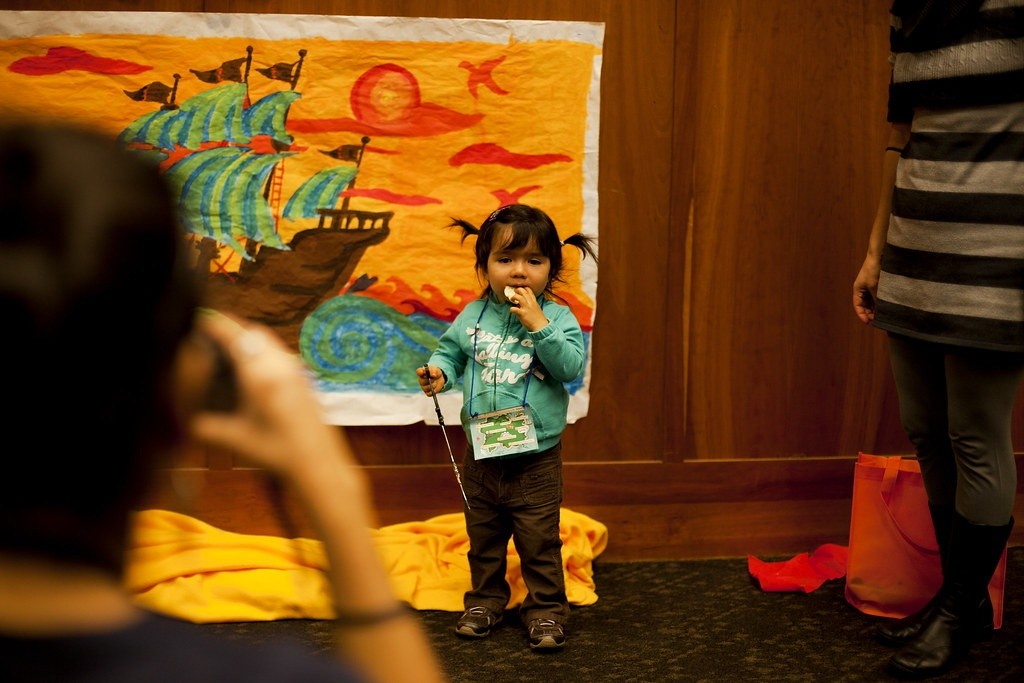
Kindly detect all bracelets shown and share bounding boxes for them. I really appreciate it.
[335,597,415,628]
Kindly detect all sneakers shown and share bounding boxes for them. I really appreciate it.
[453,604,503,637]
[528,618,564,648]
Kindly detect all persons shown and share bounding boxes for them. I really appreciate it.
[0,108,451,682]
[853,1,1024,675]
[416,205,599,651]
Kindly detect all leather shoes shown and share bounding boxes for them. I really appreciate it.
[885,594,980,680]
[878,595,994,645]
